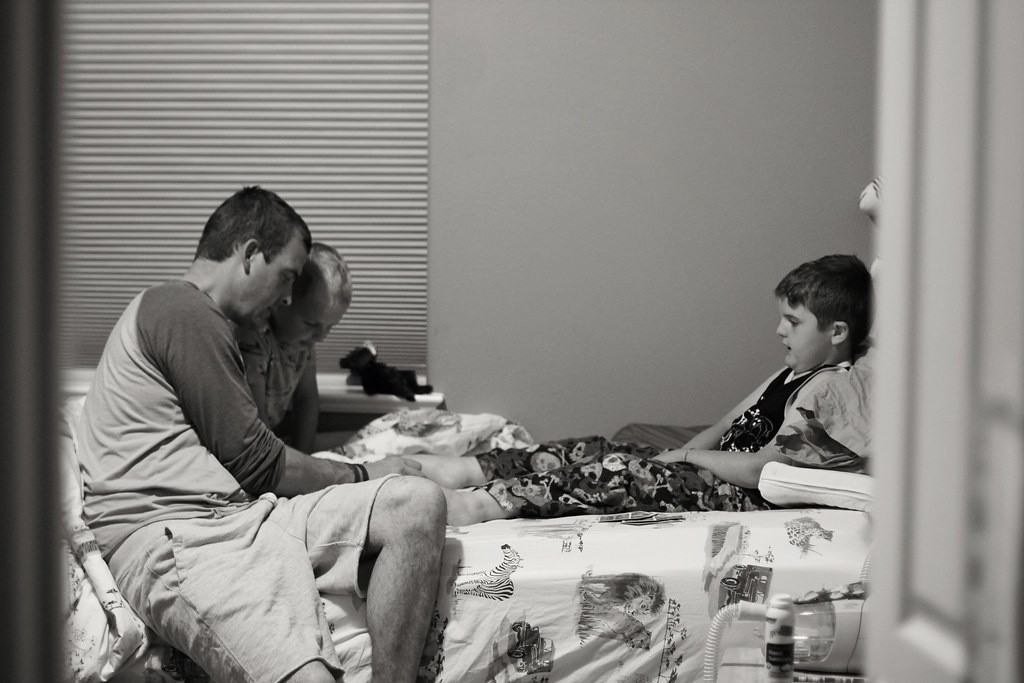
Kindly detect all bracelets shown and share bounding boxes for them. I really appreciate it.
[685,448,696,462]
[356,464,369,481]
[346,463,361,483]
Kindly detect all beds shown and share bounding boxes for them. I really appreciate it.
[320,501,868,681]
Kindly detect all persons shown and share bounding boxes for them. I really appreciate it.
[405,252,873,527]
[79,185,448,683]
[240,242,353,455]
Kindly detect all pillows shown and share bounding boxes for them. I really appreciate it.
[757,459,875,513]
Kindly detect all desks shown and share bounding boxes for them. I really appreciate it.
[308,371,447,455]
